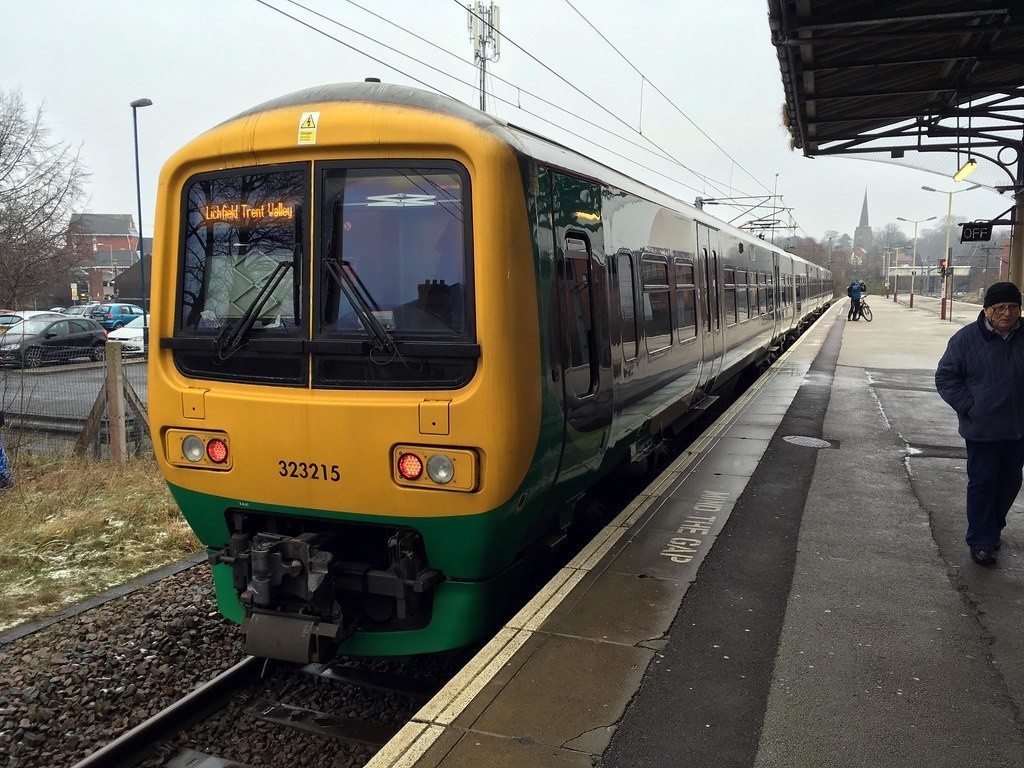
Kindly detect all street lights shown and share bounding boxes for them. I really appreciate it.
[922,184,982,320]
[880,216,938,308]
[132,97,156,360]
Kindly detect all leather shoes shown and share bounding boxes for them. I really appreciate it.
[971,538,1002,564]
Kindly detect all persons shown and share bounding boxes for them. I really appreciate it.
[936,282,1024,566]
[847,279,866,321]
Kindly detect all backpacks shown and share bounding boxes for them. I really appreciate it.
[847,283,854,297]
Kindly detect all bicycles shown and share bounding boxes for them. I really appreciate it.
[852,294,873,322]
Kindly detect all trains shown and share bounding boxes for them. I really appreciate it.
[147,76,837,690]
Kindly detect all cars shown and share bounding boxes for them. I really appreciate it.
[90,303,149,330]
[1,311,67,334]
[109,314,150,356]
[63,304,100,318]
[1,317,108,367]
[50,307,65,314]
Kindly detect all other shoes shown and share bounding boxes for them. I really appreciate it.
[848,315,857,321]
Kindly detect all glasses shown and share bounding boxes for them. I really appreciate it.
[988,305,1021,314]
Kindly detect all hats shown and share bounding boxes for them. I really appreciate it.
[984,282,1022,307]
